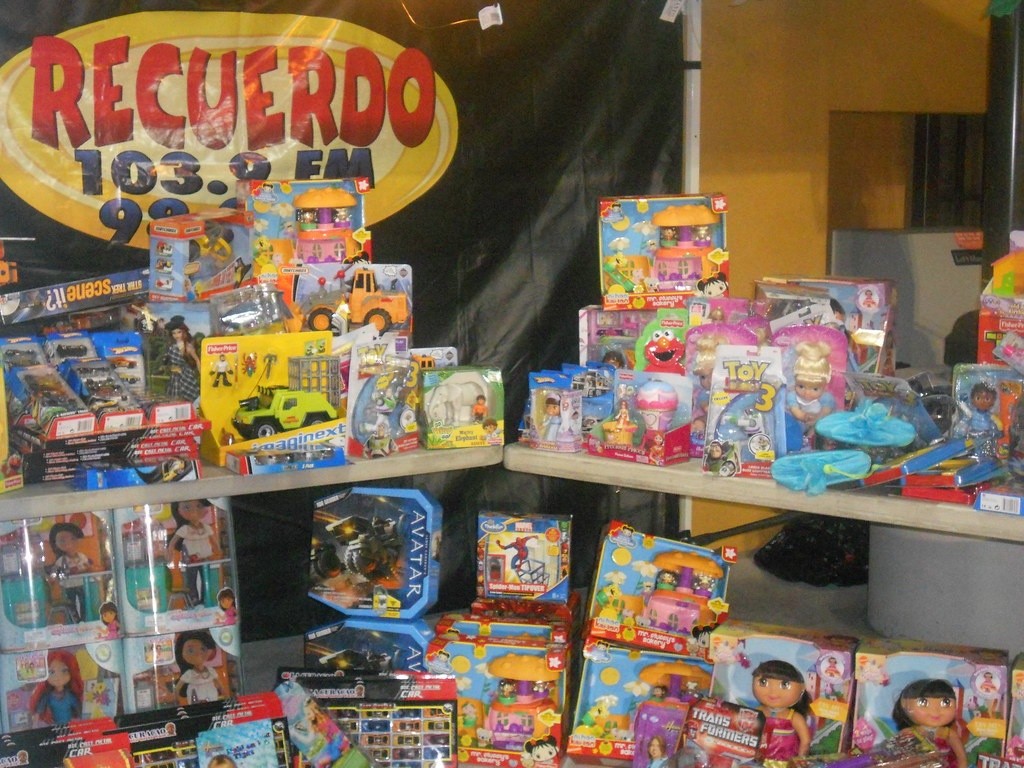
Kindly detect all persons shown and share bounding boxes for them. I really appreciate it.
[472,395,488,423]
[30,652,85,726]
[651,686,667,701]
[168,499,223,606]
[49,522,92,622]
[498,681,516,706]
[694,576,712,597]
[660,227,678,248]
[892,679,967,768]
[692,224,712,248]
[657,572,675,590]
[301,209,316,230]
[161,314,201,404]
[539,307,1024,479]
[752,660,810,768]
[334,207,351,228]
[533,680,549,697]
[171,633,225,703]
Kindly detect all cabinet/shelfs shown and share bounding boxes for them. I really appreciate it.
[0,439,1024,768]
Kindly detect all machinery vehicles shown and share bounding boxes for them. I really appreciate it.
[231,385,339,442]
[312,516,415,586]
[285,268,408,335]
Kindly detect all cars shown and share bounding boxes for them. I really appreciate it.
[134,731,287,768]
[300,704,450,768]
[4,337,139,426]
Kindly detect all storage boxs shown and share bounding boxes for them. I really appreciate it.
[0,180,1023,768]
[826,110,985,232]
